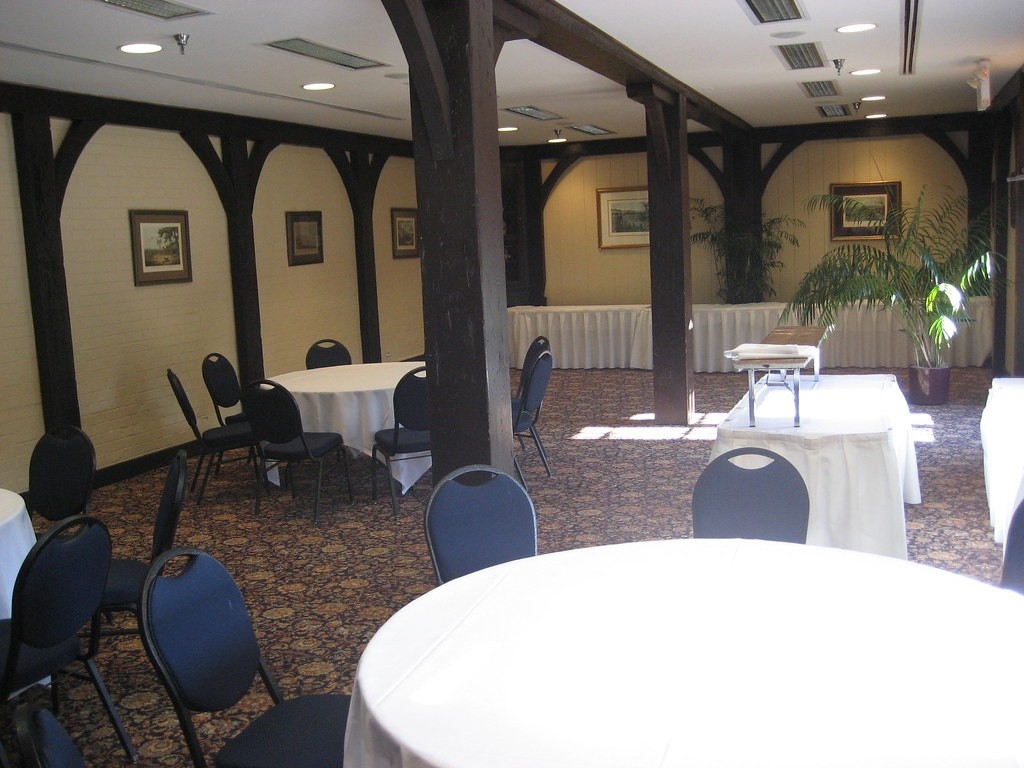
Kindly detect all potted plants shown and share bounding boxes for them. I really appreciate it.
[778,154,1014,406]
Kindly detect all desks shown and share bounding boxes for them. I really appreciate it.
[506,295,995,369]
[343,538,1024,768]
[980,378,1024,543]
[259,362,432,496]
[713,324,921,562]
[1,487,39,621]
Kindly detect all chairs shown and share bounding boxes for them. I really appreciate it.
[691,448,809,545]
[1,335,556,768]
[1000,500,1024,592]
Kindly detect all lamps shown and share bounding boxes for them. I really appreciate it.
[967,62,991,110]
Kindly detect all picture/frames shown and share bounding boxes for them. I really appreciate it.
[595,186,650,249]
[130,209,192,288]
[829,181,902,240]
[391,208,421,259]
[286,211,324,267]
